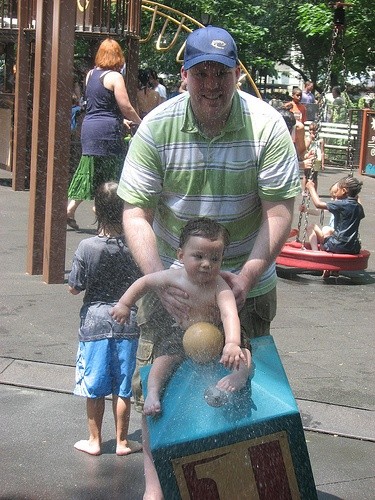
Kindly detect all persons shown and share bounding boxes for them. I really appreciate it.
[65,179,142,456]
[114,28,300,500]
[110,218,253,415]
[0,38,365,280]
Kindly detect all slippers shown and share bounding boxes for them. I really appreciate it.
[67,218,79,230]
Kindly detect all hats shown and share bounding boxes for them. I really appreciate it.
[183,25,238,71]
